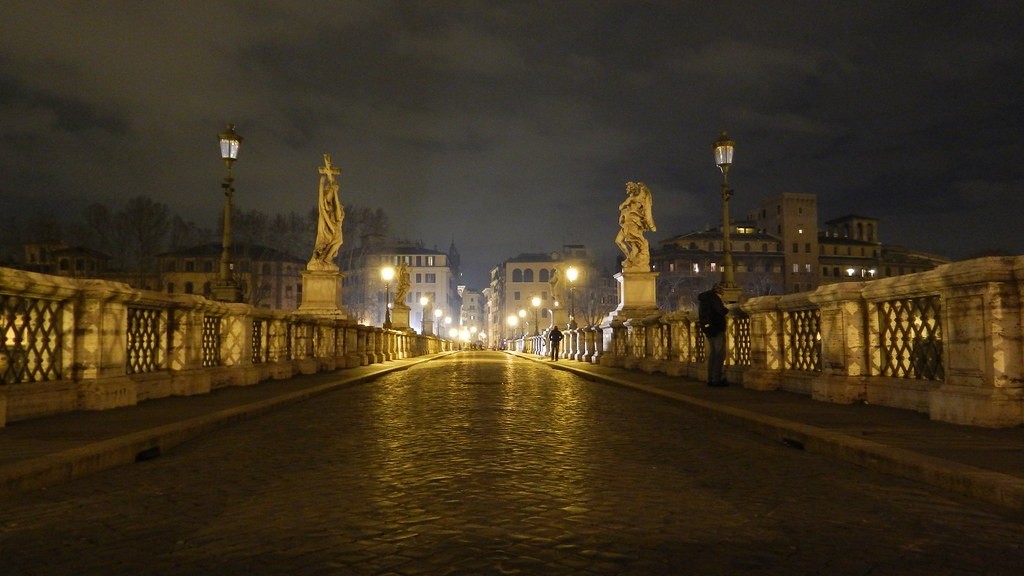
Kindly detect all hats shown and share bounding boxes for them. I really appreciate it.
[712,283,725,295]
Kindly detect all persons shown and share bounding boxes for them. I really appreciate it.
[550,267,563,305]
[549,326,562,361]
[470,341,482,350]
[615,182,641,267]
[396,268,410,304]
[309,176,345,267]
[698,285,729,387]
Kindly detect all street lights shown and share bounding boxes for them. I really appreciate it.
[532,298,541,336]
[508,317,518,339]
[435,309,442,336]
[712,130,738,288]
[420,297,428,335]
[519,309,526,335]
[567,268,577,330]
[381,267,395,330]
[216,122,244,303]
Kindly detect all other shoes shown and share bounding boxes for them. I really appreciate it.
[552,357,558,361]
[708,381,729,386]
